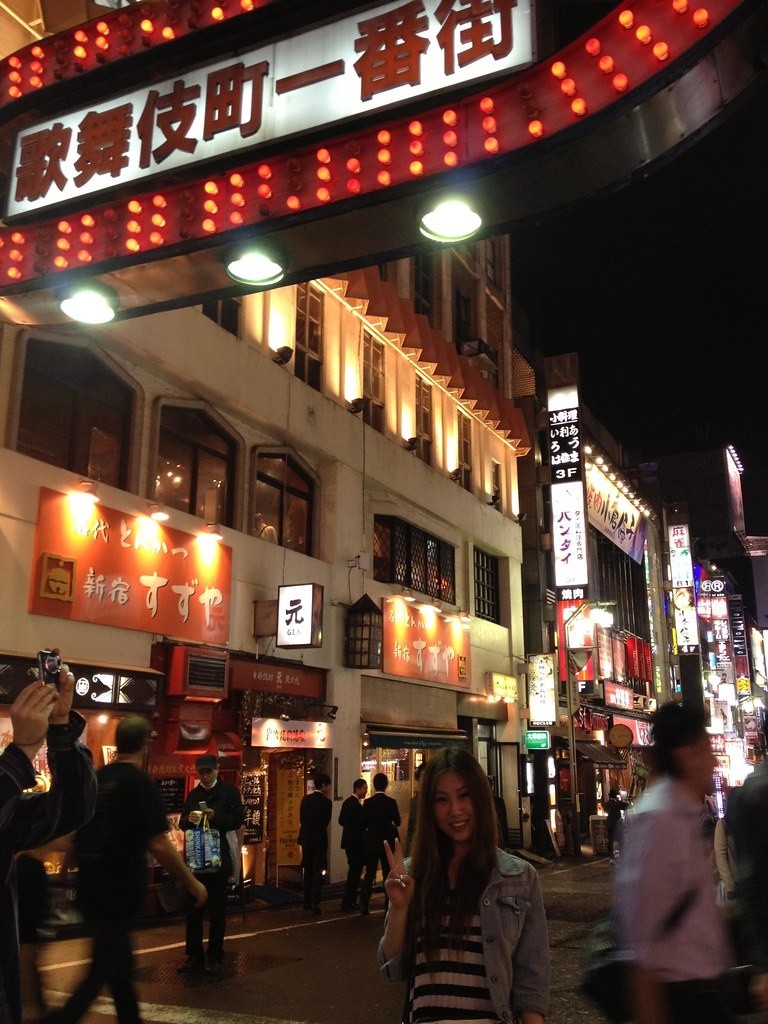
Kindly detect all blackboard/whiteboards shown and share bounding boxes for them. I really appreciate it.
[589,815,609,856]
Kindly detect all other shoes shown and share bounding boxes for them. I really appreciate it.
[302,903,322,914]
[203,951,224,975]
[358,905,370,915]
[178,955,202,977]
[341,900,357,911]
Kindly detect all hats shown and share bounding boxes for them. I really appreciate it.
[609,789,618,795]
[196,755,217,771]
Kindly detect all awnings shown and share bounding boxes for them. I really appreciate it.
[562,736,628,770]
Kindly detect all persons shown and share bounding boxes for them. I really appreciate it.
[603,789,630,865]
[0,648,98,1024]
[674,588,694,610]
[705,789,719,822]
[339,778,368,911]
[714,786,740,899]
[358,773,401,913]
[738,710,768,1024]
[179,755,244,973]
[616,705,732,1024]
[55,714,207,1024]
[296,773,333,912]
[721,673,726,683]
[377,746,551,1024]
[255,513,278,544]
[404,763,428,860]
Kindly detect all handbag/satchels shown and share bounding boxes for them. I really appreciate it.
[183,814,222,875]
[579,929,630,1024]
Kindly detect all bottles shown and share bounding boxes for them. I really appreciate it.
[211,849,220,867]
[186,830,194,851]
[164,813,184,852]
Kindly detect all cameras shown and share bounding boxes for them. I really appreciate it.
[37,649,62,694]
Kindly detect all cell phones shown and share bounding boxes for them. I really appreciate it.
[199,801,207,809]
